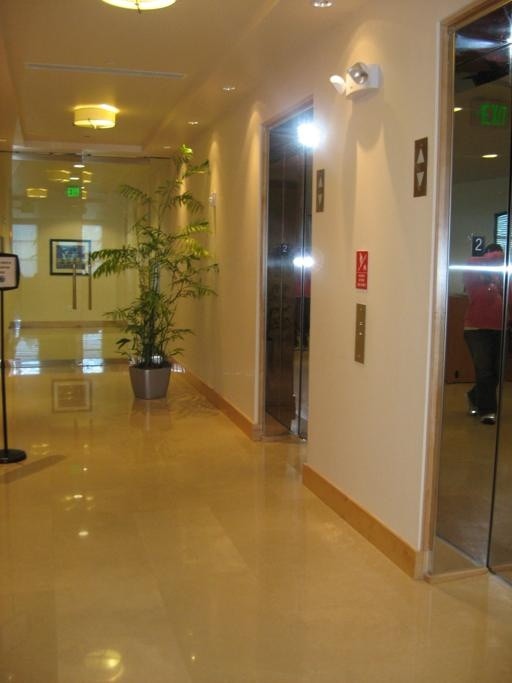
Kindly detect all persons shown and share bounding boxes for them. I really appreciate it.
[291,268,310,353]
[459,241,506,423]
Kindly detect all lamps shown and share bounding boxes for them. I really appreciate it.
[25,187,48,199]
[73,104,116,129]
[48,169,70,185]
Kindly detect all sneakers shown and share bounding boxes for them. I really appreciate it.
[469,403,479,415]
[480,414,496,425]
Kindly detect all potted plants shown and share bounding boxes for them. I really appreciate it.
[86,144,216,400]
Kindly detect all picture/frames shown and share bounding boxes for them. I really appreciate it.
[49,239,91,276]
[52,379,93,413]
